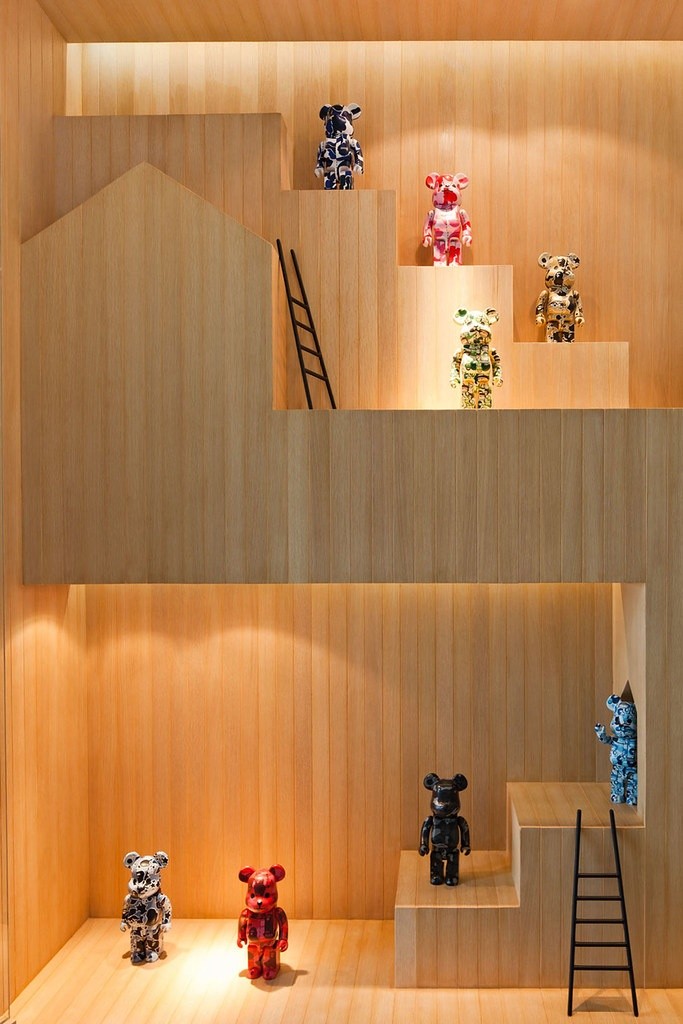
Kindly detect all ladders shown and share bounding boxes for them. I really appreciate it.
[567,810,640,1019]
[274,238,337,411]
